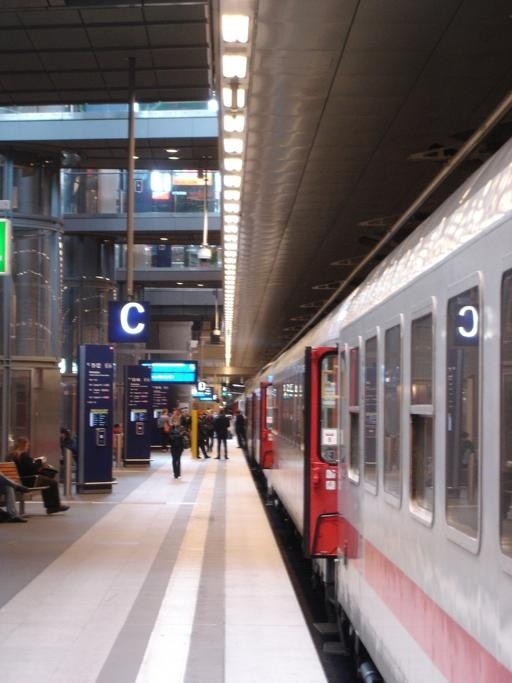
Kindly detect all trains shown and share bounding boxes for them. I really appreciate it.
[231,136,512,683]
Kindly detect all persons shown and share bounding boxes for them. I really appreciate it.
[113,423,121,434]
[168,415,185,479]
[7,436,70,513]
[59,428,78,460]
[0,484,28,523]
[159,405,236,460]
[233,408,247,449]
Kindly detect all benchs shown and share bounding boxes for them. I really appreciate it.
[0,462,50,517]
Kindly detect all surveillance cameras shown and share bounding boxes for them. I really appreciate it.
[213,329,221,338]
[197,248,211,262]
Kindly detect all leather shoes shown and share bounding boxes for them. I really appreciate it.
[12,516,27,522]
[46,504,69,513]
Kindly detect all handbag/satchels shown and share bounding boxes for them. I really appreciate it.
[182,436,192,448]
[39,461,58,476]
